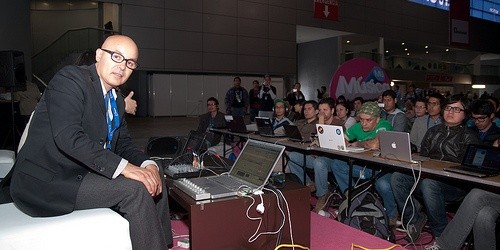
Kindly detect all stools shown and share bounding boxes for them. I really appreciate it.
[0,150,16,178]
[0,201,133,250]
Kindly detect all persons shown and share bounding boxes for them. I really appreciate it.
[194,74,500,250]
[11,34,175,250]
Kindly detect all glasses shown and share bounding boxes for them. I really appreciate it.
[472,116,487,123]
[102,48,139,70]
[443,106,464,113]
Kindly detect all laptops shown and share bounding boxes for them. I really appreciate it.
[281,123,315,143]
[254,116,287,137]
[378,130,431,163]
[187,139,286,199]
[158,130,206,164]
[314,124,364,152]
[443,143,500,178]
[232,115,256,133]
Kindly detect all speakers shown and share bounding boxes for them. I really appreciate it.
[0,49,28,94]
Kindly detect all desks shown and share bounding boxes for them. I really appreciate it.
[165,155,311,250]
[211,127,500,217]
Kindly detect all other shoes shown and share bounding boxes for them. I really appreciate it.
[315,194,329,212]
[408,213,428,242]
[308,181,316,192]
